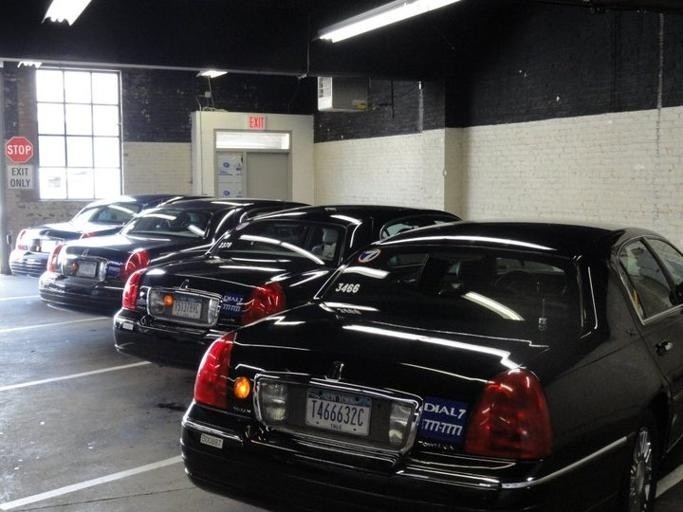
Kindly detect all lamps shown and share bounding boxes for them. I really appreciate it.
[16,60,42,69]
[196,70,228,79]
[313,0,461,45]
[43,0,92,26]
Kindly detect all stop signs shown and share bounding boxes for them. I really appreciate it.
[5,136,33,164]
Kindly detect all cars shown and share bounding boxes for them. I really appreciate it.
[8,195,178,278]
[38,198,309,312]
[178,221,682,510]
[113,205,462,369]
